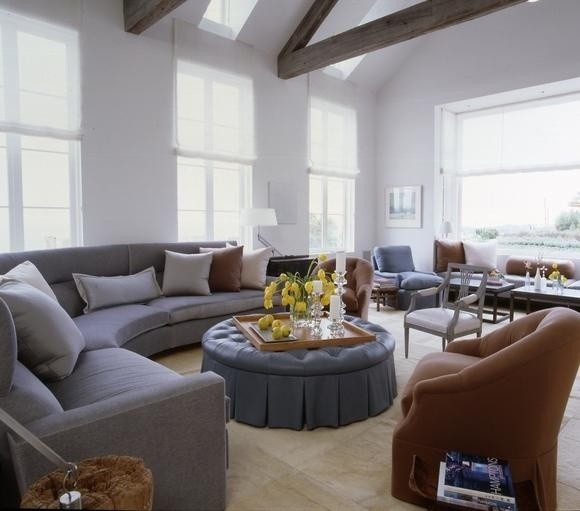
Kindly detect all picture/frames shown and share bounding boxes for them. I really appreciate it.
[386,186,422,228]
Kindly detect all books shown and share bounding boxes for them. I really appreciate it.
[443,447,516,510]
[435,460,517,511]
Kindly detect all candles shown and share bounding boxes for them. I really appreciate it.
[329,294,341,319]
[336,250,346,273]
[312,280,322,291]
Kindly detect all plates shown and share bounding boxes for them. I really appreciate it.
[250,320,299,343]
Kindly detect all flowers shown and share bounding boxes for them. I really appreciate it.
[263,253,333,318]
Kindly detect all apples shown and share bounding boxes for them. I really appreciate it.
[258,318,269,329]
[271,327,282,340]
[265,315,274,325]
[271,319,282,328]
[282,325,291,337]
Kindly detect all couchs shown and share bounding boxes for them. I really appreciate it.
[0,241,287,509]
[434,253,580,317]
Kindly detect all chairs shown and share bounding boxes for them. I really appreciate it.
[392,305,580,511]
[308,254,374,321]
[403,261,489,359]
[367,247,443,312]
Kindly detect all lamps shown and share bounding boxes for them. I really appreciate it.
[240,208,284,257]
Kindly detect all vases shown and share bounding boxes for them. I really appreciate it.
[289,301,313,329]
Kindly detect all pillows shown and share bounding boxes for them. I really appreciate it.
[0,258,86,384]
[505,259,575,281]
[72,267,163,314]
[432,238,499,271]
[160,242,274,296]
